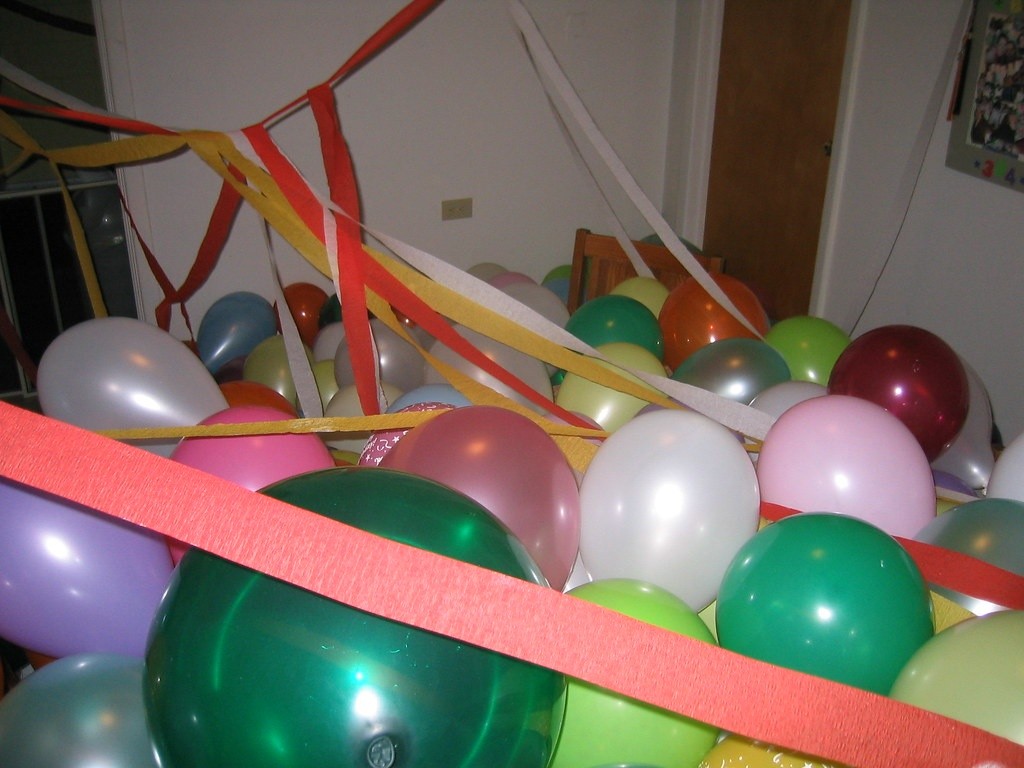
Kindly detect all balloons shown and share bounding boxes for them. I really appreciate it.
[0,233,1024,768]
[148,467,569,768]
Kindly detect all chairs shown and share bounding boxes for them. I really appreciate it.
[564,227,725,319]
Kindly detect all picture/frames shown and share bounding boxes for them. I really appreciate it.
[945,0,1024,192]
